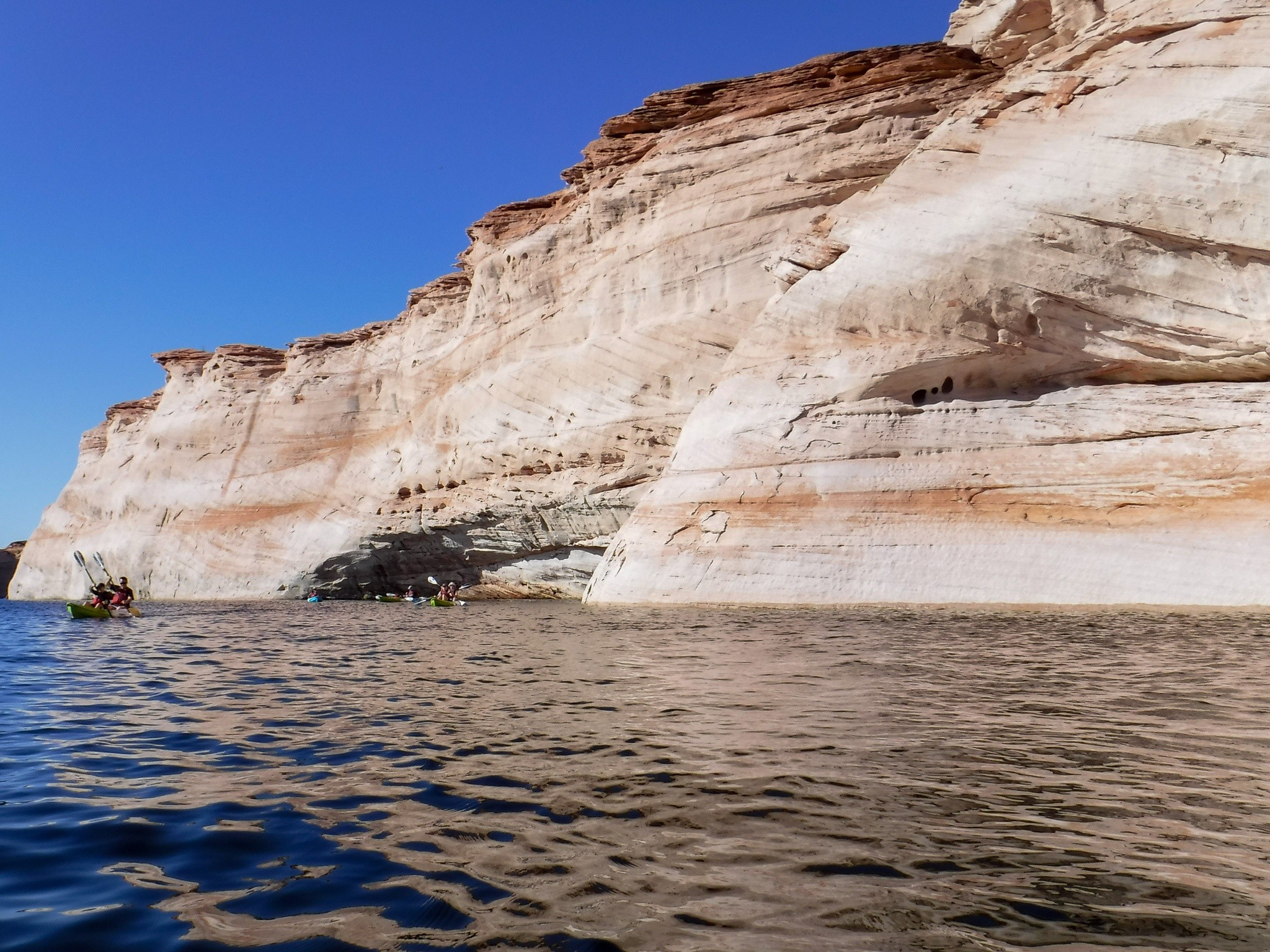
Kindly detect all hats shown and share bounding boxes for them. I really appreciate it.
[448,582,456,587]
[407,585,415,590]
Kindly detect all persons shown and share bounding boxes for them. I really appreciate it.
[83,582,111,609]
[447,582,458,602]
[105,576,134,610]
[406,585,414,599]
[307,587,319,598]
[437,583,451,601]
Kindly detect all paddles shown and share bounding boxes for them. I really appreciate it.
[92,551,141,618]
[427,575,467,606]
[375,595,383,600]
[74,550,113,618]
[385,592,414,601]
[414,582,472,605]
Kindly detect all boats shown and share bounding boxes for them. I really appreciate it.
[430,598,455,607]
[307,596,323,603]
[377,596,409,602]
[65,600,141,620]
